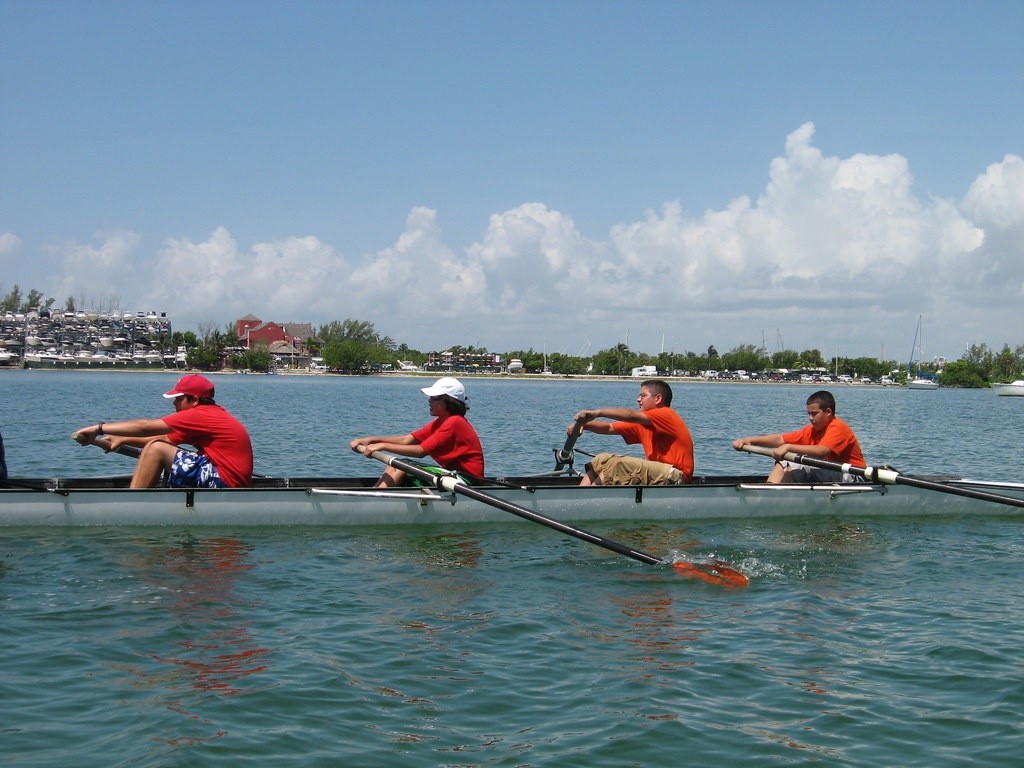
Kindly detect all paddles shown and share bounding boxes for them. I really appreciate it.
[355,443,750,590]
[551,415,587,477]
[70,430,267,478]
[732,440,1024,508]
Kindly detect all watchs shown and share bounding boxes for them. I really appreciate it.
[98,422,105,435]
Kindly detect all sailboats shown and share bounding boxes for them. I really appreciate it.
[906,314,939,390]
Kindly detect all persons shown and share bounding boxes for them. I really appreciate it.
[566,380,694,485]
[76,374,253,489]
[0,433,8,481]
[351,378,484,487]
[734,390,869,482]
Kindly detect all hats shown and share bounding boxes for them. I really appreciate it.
[162,374,214,399]
[421,378,467,405]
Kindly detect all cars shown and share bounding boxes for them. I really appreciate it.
[700,370,836,382]
[859,376,871,383]
[837,374,853,383]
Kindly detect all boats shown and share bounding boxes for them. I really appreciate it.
[989,380,1024,396]
[0,311,175,361]
[0,475,1024,525]
[458,353,493,367]
[508,359,523,371]
